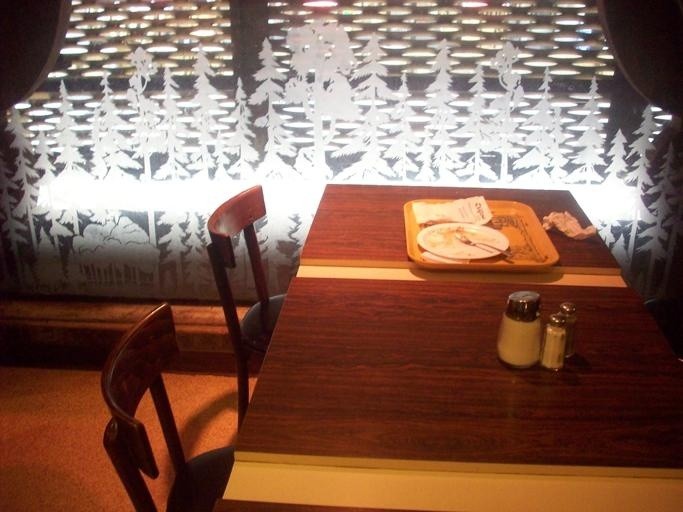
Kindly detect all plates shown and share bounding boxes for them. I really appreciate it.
[415,221,509,259]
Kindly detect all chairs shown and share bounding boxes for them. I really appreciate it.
[101,302,235,512]
[207,183,284,438]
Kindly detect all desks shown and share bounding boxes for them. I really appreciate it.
[222,182,683,511]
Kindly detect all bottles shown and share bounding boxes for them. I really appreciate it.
[541,301,575,372]
[495,290,541,370]
